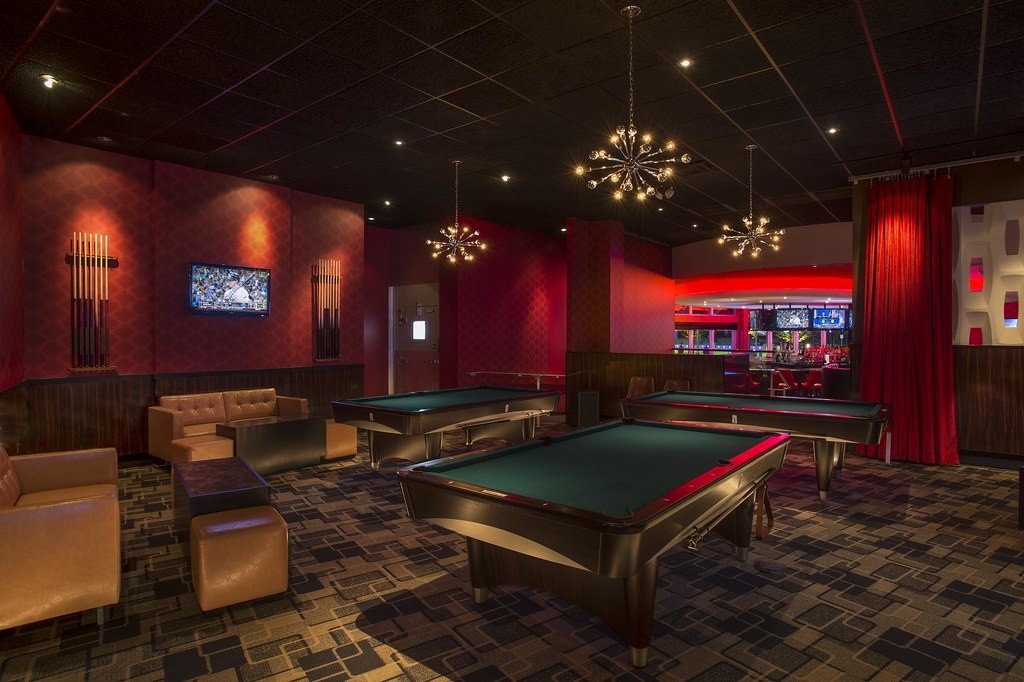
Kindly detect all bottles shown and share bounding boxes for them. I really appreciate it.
[799,344,846,364]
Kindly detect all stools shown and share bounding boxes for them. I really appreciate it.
[171,435,234,490]
[190,505,290,611]
[322,418,357,461]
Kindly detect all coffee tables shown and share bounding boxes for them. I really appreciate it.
[173,456,271,566]
[214,414,327,476]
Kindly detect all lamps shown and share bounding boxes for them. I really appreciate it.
[423,159,486,266]
[574,5,693,201]
[718,144,786,257]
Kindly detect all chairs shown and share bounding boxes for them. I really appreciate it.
[618,376,653,421]
[664,380,690,393]
[745,369,820,397]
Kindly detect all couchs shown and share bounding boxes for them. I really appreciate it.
[0,445,122,631]
[148,388,308,466]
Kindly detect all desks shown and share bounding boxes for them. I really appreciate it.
[620,390,889,502]
[331,385,561,470]
[397,415,792,667]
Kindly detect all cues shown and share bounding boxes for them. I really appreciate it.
[317,258,341,359]
[72,232,110,368]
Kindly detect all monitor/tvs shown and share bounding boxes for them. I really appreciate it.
[812,308,847,330]
[775,308,811,330]
[186,262,271,317]
[750,309,775,331]
